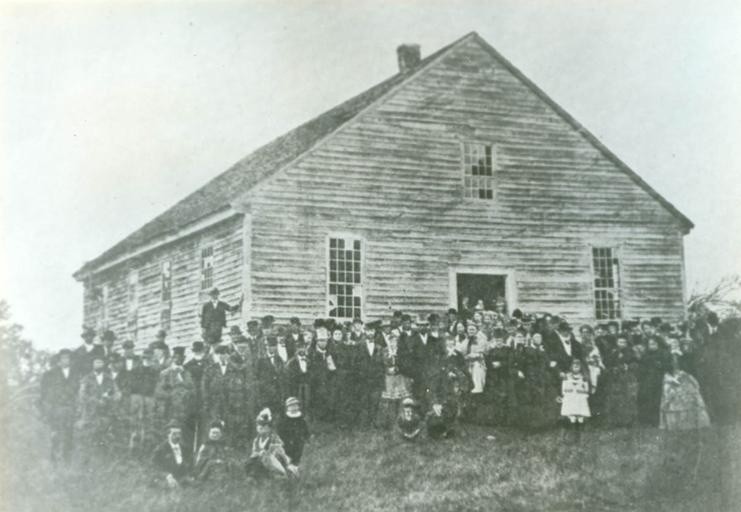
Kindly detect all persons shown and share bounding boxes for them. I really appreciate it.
[247,294,741,432]
[38,288,310,489]
